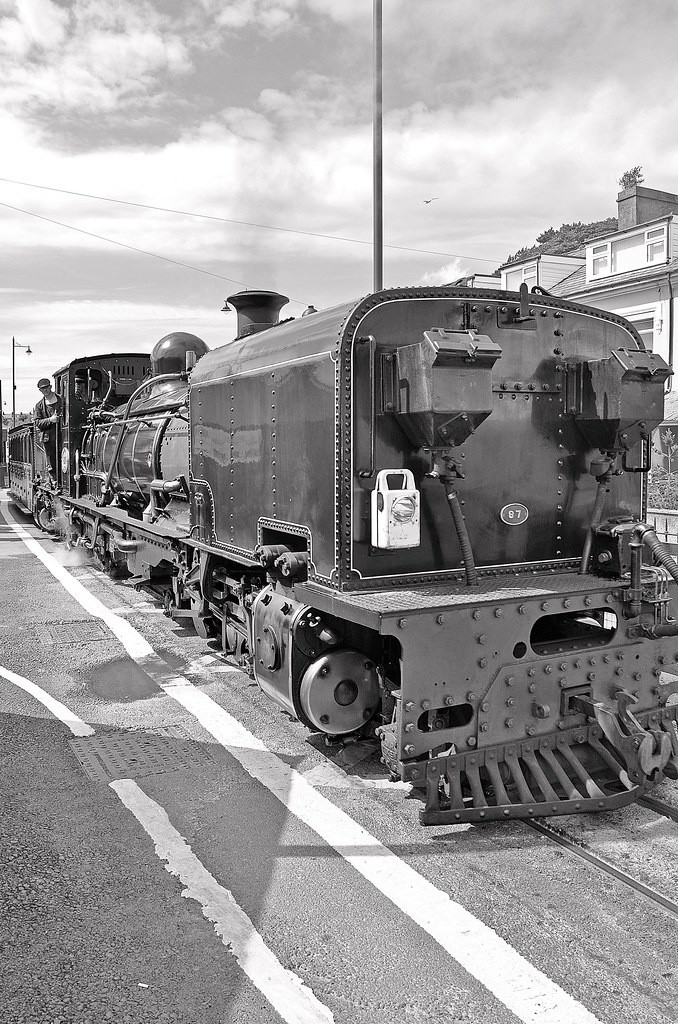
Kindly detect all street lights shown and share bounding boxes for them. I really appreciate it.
[12,336,33,428]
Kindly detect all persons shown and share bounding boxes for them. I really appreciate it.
[33,379,67,490]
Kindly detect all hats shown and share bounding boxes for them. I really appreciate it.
[37,379,50,389]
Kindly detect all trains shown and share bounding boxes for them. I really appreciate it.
[8,283,678,827]
